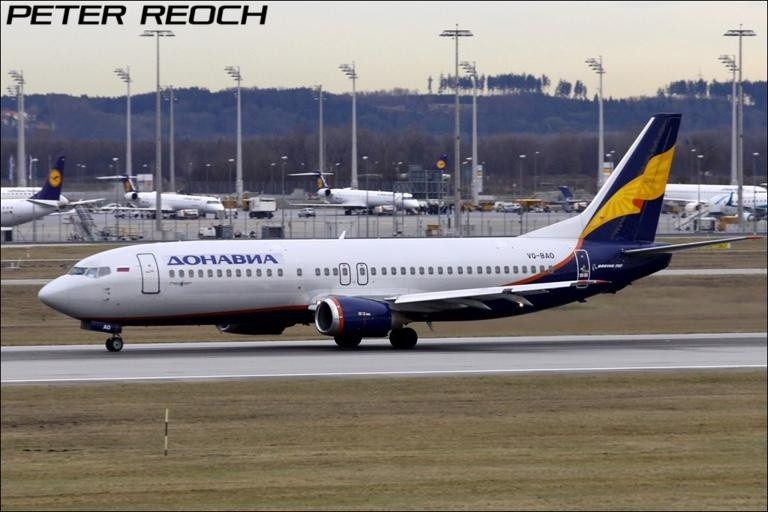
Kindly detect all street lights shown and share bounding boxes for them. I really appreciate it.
[7,24,760,235]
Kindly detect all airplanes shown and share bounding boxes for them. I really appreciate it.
[597,162,767,231]
[1,156,106,241]
[37,114,767,352]
